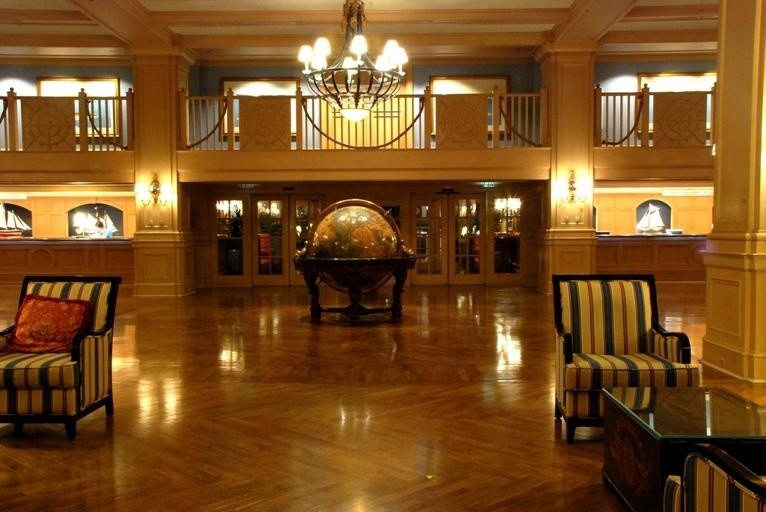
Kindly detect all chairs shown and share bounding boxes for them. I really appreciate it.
[547,274,699,443]
[659,444,765,512]
[1,275,120,440]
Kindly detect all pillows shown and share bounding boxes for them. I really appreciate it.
[8,292,93,356]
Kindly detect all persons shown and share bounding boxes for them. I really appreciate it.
[94,221,113,238]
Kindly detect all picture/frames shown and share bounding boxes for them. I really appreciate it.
[216,76,302,141]
[636,69,720,138]
[36,76,121,138]
[426,74,511,138]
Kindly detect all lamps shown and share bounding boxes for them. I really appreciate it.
[555,167,589,210]
[298,0,409,124]
[133,171,179,210]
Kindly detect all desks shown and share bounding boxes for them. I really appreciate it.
[602,387,765,512]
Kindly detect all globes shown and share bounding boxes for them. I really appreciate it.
[292,198,417,327]
